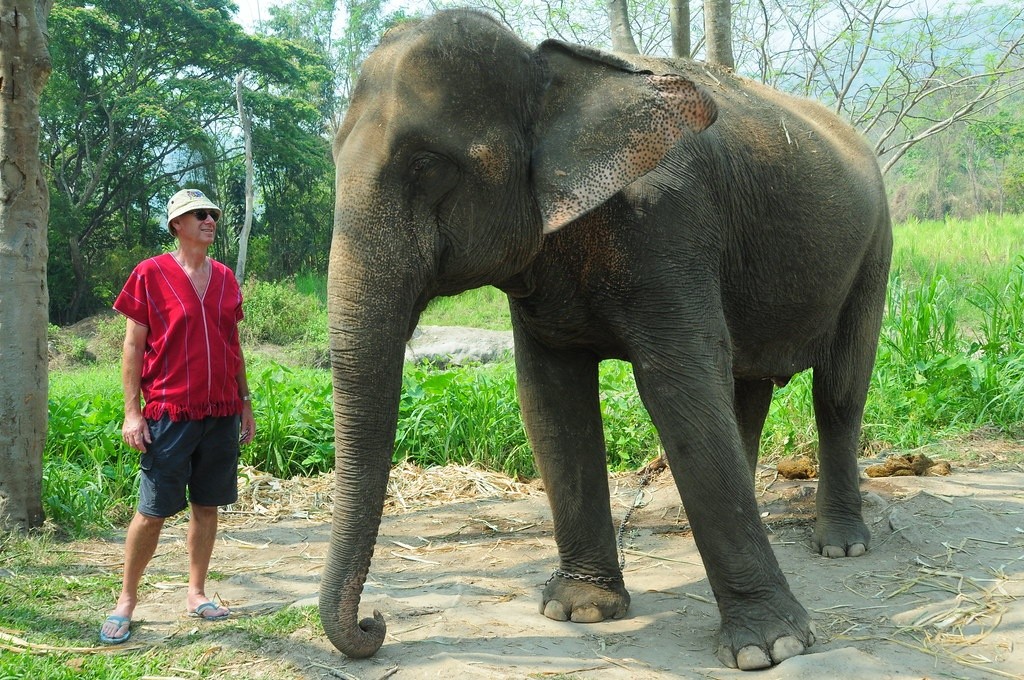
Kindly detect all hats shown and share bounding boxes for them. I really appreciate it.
[167,189,222,237]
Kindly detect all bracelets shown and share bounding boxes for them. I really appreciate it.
[243,396,251,400]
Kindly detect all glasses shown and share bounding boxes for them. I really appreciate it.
[184,208,219,222]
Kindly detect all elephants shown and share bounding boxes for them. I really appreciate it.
[316,6,893,672]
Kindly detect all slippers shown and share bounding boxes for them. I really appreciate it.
[187,602,230,620]
[100,614,131,644]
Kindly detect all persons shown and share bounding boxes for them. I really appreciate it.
[99,189,256,644]
